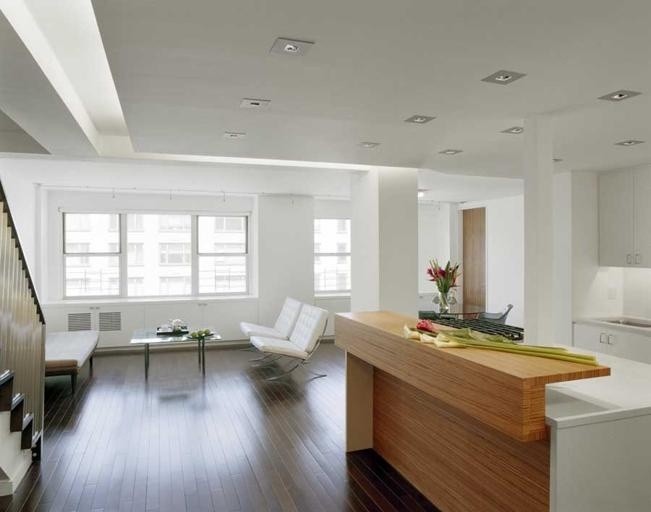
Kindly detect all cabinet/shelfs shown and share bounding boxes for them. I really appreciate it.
[573,323,651,365]
[598,163,651,268]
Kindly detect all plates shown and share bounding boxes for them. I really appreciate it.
[188,332,214,340]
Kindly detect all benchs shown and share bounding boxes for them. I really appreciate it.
[45,329,99,395]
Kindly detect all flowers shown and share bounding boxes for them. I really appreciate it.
[427,257,463,312]
[402,318,599,366]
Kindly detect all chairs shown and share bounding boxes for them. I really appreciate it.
[239,296,330,381]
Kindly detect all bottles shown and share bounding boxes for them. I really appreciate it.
[172,318,182,331]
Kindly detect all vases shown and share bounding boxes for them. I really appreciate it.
[439,292,450,312]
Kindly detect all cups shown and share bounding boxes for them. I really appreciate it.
[159,324,170,331]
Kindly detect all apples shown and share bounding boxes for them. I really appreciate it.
[191,329,210,337]
